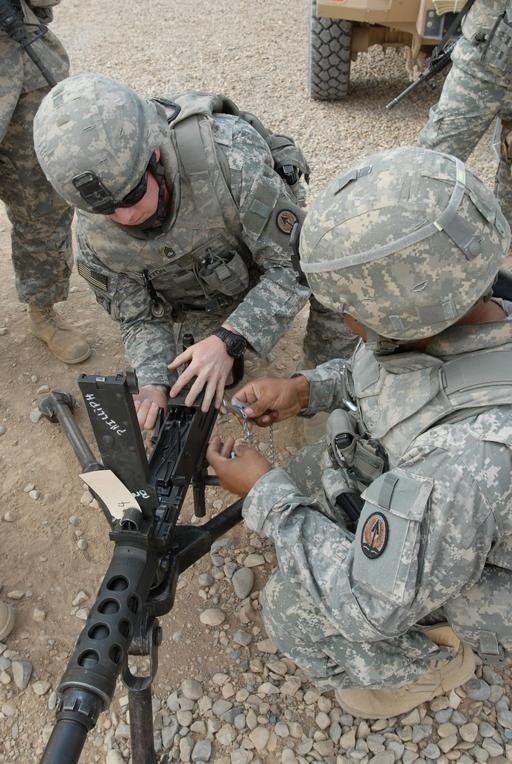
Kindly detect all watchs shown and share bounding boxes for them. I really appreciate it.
[211,326,248,362]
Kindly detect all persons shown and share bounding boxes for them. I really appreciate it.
[0,1,95,367]
[0,596,17,646]
[29,70,314,435]
[402,1,511,238]
[203,143,511,725]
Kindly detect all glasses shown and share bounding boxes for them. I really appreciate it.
[89,173,150,215]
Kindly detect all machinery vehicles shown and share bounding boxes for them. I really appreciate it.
[308,1,472,102]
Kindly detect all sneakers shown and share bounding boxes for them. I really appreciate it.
[28,303,92,364]
[334,622,477,721]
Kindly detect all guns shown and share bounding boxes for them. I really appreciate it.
[385,36,459,111]
[37,333,247,764]
[0,0,58,86]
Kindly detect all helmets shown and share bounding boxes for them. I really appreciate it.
[32,72,171,216]
[298,145,511,342]
